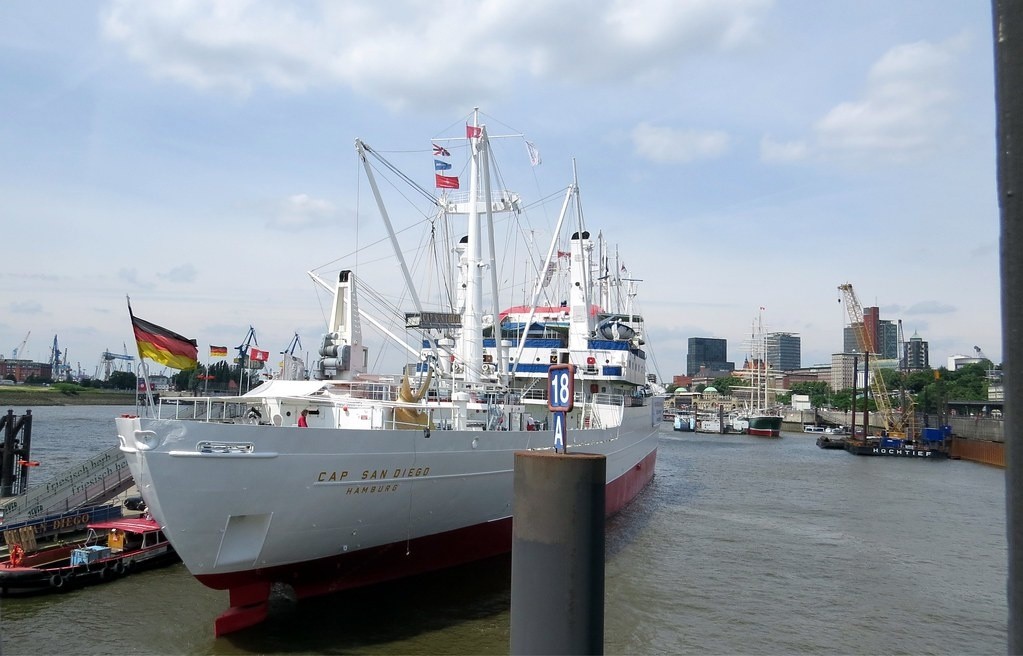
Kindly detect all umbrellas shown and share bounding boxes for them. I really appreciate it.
[675,388,688,393]
[704,387,717,393]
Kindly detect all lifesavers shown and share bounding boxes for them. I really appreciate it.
[114,562,126,576]
[50,574,64,588]
[66,573,76,584]
[127,559,138,571]
[76,574,87,586]
[9,547,25,565]
[100,566,113,580]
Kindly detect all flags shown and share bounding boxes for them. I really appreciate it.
[436,174,459,189]
[132,316,198,370]
[432,143,450,156]
[434,159,451,170]
[526,141,542,167]
[210,345,227,356]
[250,349,269,362]
[467,126,481,137]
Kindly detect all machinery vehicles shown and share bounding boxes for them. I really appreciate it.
[837,280,921,447]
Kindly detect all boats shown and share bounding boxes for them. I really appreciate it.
[123,105,660,620]
[804,425,864,436]
[674,305,786,438]
[0,507,179,599]
[599,322,636,340]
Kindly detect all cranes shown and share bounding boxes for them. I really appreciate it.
[12,330,134,387]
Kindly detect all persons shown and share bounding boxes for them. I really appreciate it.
[298,409,308,427]
[527,417,536,431]
